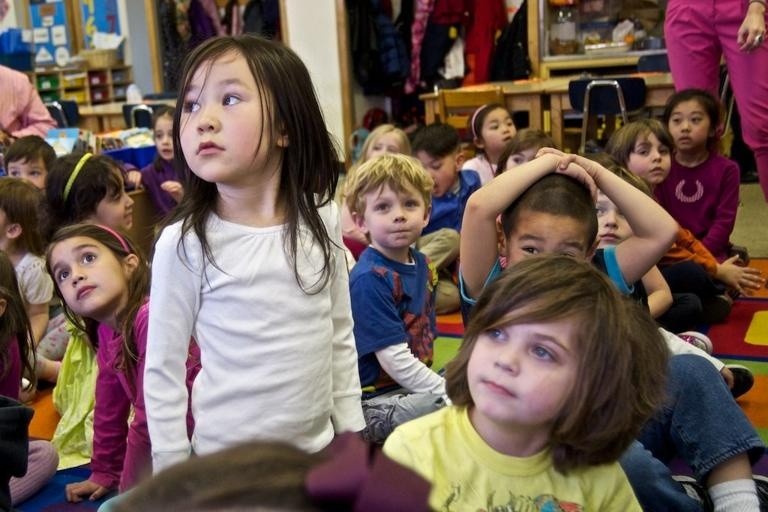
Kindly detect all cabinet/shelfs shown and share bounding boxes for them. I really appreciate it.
[27,67,91,108]
[334,0,528,173]
[525,0,729,77]
[88,64,135,106]
[145,0,291,95]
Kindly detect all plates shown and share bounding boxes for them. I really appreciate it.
[585,42,628,54]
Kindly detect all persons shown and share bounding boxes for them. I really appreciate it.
[141,31,374,478]
[1,101,767,511]
[1,66,61,150]
[656,87,740,265]
[661,0,767,206]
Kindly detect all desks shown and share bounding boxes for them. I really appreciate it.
[77,99,178,134]
[419,76,572,133]
[545,73,676,151]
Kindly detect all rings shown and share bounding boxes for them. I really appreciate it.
[754,34,762,46]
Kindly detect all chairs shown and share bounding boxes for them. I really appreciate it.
[563,77,648,157]
[440,87,506,129]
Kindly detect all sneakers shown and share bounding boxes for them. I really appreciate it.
[678,331,713,357]
[720,364,755,401]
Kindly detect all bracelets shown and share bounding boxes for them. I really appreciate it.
[748,1,767,9]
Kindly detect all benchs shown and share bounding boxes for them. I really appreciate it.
[102,144,159,170]
[124,188,167,263]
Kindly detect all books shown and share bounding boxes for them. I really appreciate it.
[576,41,632,55]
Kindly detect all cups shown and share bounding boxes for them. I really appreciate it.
[640,37,663,49]
